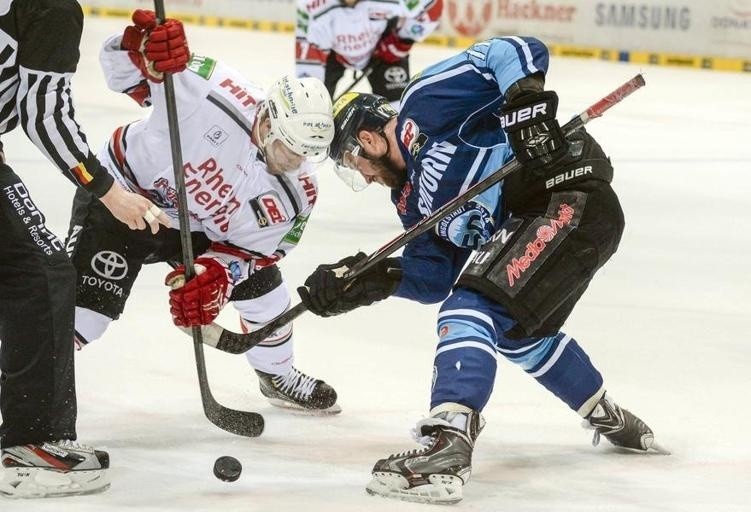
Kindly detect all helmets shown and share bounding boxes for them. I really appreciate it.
[258,78,334,177]
[331,93,396,192]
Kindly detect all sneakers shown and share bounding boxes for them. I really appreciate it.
[256,367,336,409]
[2,442,110,471]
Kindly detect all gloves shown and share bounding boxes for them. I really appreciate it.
[494,91,569,176]
[121,10,187,85]
[298,251,402,316]
[166,259,235,327]
[374,34,413,64]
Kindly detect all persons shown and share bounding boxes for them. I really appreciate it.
[296,34,670,489]
[294,1,444,112]
[1,1,173,474]
[64,9,342,416]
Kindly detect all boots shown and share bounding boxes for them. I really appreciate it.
[373,411,486,483]
[583,394,652,450]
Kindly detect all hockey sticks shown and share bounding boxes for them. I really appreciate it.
[155,0,263,438]
[172,74,645,355]
